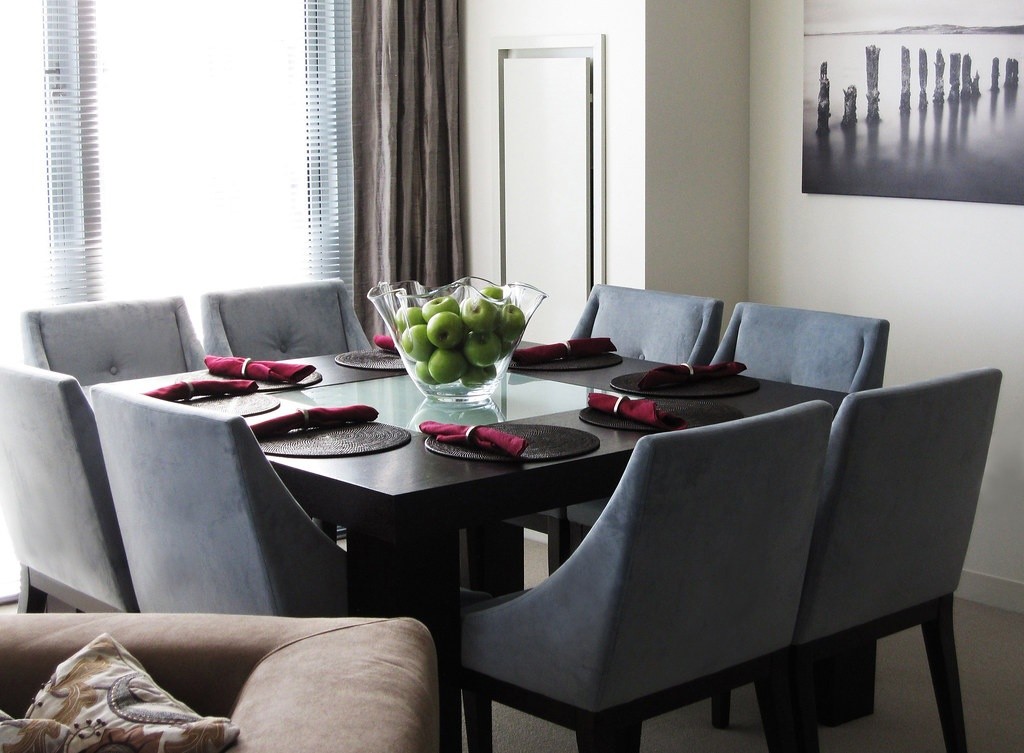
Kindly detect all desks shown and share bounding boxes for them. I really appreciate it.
[81,349,853,753]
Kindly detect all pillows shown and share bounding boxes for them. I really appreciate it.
[0,632,239,753]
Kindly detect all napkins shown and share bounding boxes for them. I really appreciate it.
[637,359,748,389]
[512,336,617,365]
[373,334,401,353]
[144,380,261,400]
[250,405,377,439]
[587,389,687,428]
[203,350,315,385]
[418,420,530,459]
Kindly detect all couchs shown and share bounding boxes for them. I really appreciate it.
[0,609,443,753]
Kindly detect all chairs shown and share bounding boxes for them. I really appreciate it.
[91,385,372,614]
[706,367,1003,753]
[21,292,208,396]
[706,301,891,396]
[200,274,377,371]
[473,271,722,588]
[0,366,146,616]
[446,399,837,752]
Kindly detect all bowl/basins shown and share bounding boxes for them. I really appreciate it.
[367,276,548,407]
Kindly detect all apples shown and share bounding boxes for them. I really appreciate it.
[394,286,525,389]
